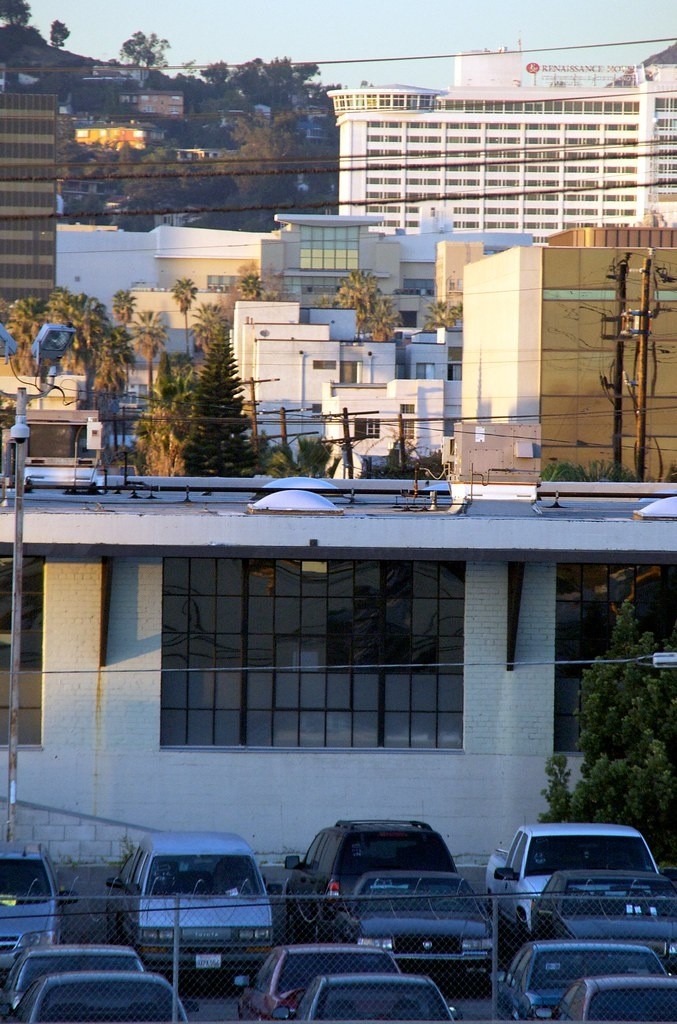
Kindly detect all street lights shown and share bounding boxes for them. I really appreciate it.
[0,321,78,850]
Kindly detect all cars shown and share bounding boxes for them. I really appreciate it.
[0,944,149,1020]
[1,969,199,1024]
[535,869,677,973]
[270,970,463,1023]
[235,943,403,1021]
[534,972,677,1024]
[491,939,672,1022]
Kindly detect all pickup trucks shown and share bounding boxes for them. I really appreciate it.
[484,822,677,950]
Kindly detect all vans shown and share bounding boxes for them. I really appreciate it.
[104,831,283,990]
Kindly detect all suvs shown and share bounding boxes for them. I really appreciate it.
[282,819,461,949]
[0,841,80,975]
